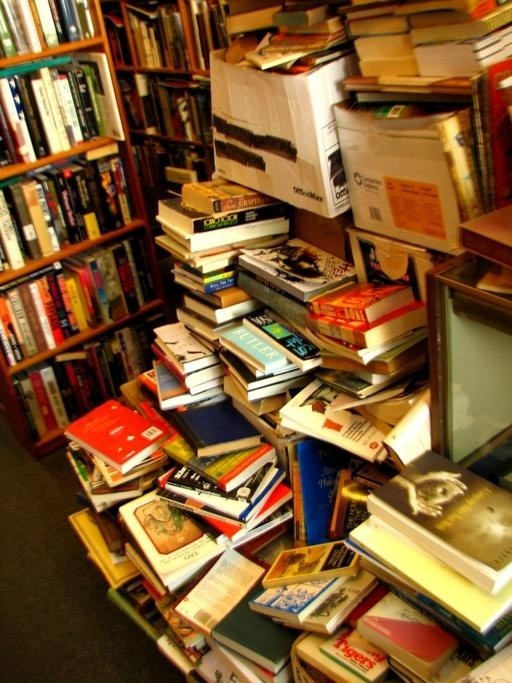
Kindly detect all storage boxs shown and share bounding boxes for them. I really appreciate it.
[347,224,437,302]
[210,48,359,219]
[332,99,482,258]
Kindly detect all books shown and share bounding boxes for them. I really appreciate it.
[105,1,510,213]
[0,143,131,274]
[12,310,174,445]
[2,234,157,369]
[2,51,125,166]
[62,177,510,683]
[1,1,96,61]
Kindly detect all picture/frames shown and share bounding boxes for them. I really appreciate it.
[426,254,511,473]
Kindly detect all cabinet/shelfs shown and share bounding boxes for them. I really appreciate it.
[99,0,232,269]
[1,0,170,463]
